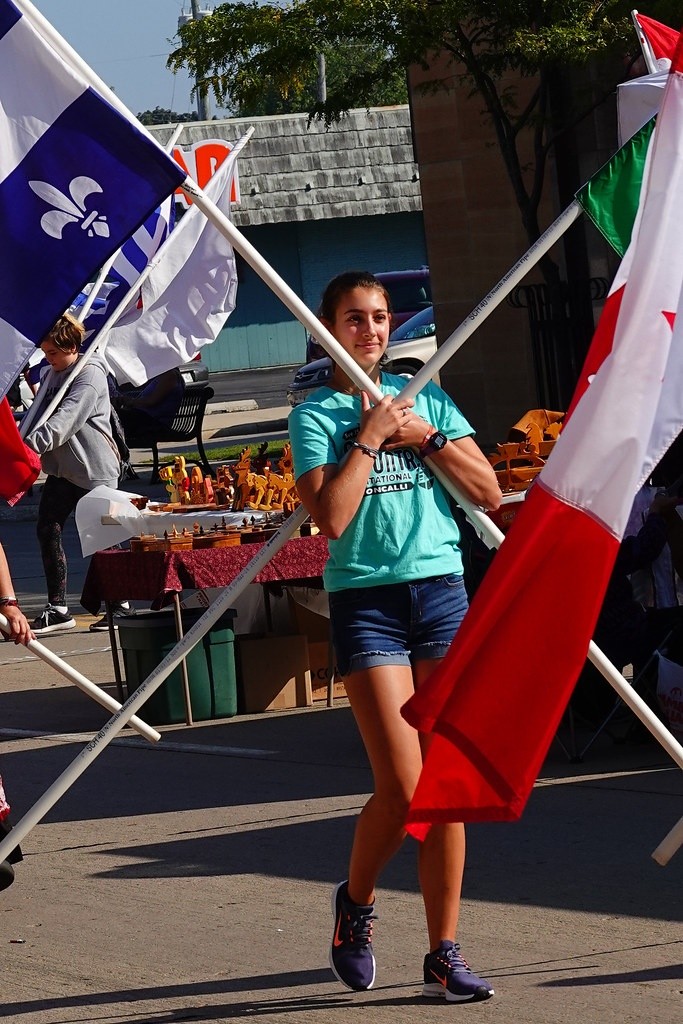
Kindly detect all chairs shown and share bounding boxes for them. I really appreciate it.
[555,605,683,763]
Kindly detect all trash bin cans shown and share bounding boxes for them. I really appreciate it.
[112,606,238,721]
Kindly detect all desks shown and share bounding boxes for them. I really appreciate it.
[78,500,526,726]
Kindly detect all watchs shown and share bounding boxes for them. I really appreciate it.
[419,430,447,458]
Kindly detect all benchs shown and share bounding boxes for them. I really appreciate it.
[125,381,216,485]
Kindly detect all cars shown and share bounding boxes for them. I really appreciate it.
[305,267,431,364]
[287,307,442,408]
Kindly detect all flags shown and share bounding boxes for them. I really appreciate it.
[398,8,683,844]
[0,0,238,507]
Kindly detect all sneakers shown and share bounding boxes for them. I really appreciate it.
[328,880,379,992]
[89,605,136,632]
[422,939,495,1004]
[28,602,77,634]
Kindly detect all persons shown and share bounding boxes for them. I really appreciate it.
[0,542,38,891]
[27,314,139,634]
[287,270,504,1002]
[5,360,37,411]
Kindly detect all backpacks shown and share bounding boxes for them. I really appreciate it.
[86,401,130,464]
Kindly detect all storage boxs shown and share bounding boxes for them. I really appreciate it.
[113,587,349,725]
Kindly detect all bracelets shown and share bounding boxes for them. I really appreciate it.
[1,596,20,608]
[352,441,379,457]
[420,424,434,447]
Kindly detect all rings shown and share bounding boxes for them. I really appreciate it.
[401,409,406,416]
